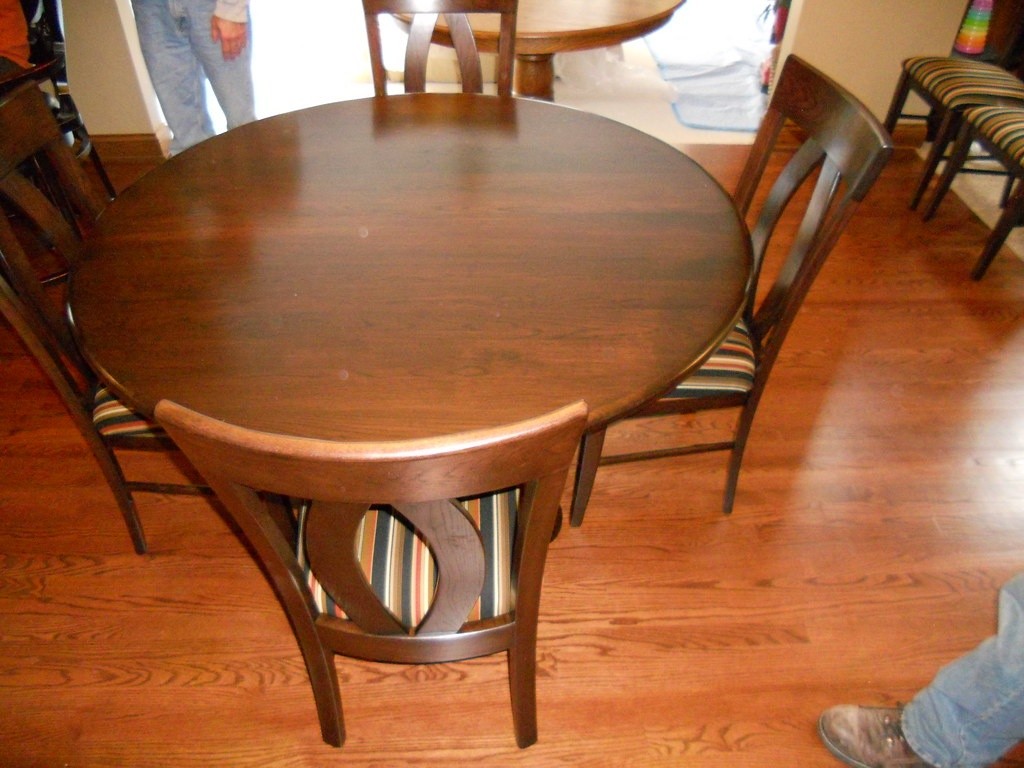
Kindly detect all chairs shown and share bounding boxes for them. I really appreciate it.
[921,104,1024,281]
[362,0,517,98]
[567,55,895,531]
[0,83,303,558]
[881,0,1024,213]
[0,51,118,287]
[155,395,590,744]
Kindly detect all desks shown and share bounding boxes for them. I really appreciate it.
[368,0,682,105]
[68,94,755,514]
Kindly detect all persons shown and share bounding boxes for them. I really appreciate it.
[816,571,1024,768]
[0,0,31,73]
[130,0,258,158]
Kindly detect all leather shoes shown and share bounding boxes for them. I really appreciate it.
[818,704,932,768]
